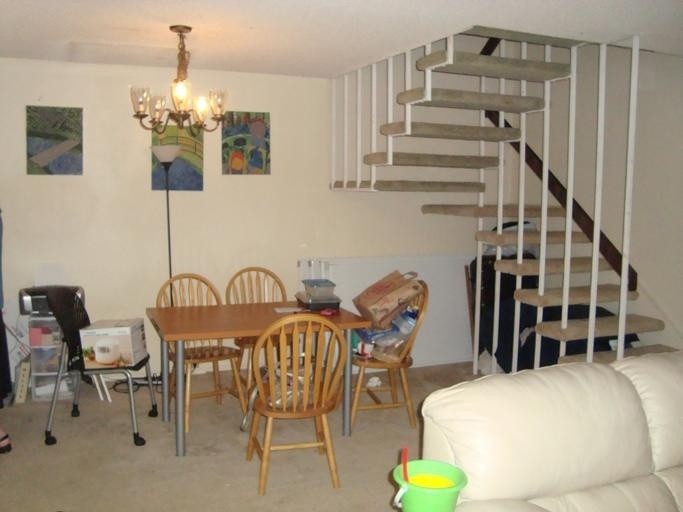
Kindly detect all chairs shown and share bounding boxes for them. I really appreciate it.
[45,286,159,446]
[226,267,300,393]
[156,273,247,433]
[351,280,428,428]
[245,312,347,494]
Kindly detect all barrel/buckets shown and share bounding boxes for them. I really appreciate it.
[392,460,468,511]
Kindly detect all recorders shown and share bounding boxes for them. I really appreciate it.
[18,286,86,316]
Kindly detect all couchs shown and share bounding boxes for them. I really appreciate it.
[421,352,683,512]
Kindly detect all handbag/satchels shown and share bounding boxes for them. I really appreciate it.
[352,271,424,330]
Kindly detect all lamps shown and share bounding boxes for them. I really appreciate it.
[129,25,227,136]
[149,146,182,306]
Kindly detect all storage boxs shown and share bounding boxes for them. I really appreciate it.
[79,318,147,370]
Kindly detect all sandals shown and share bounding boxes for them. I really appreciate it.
[0,434,13,453]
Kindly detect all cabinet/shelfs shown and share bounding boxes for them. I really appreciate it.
[27,314,74,401]
